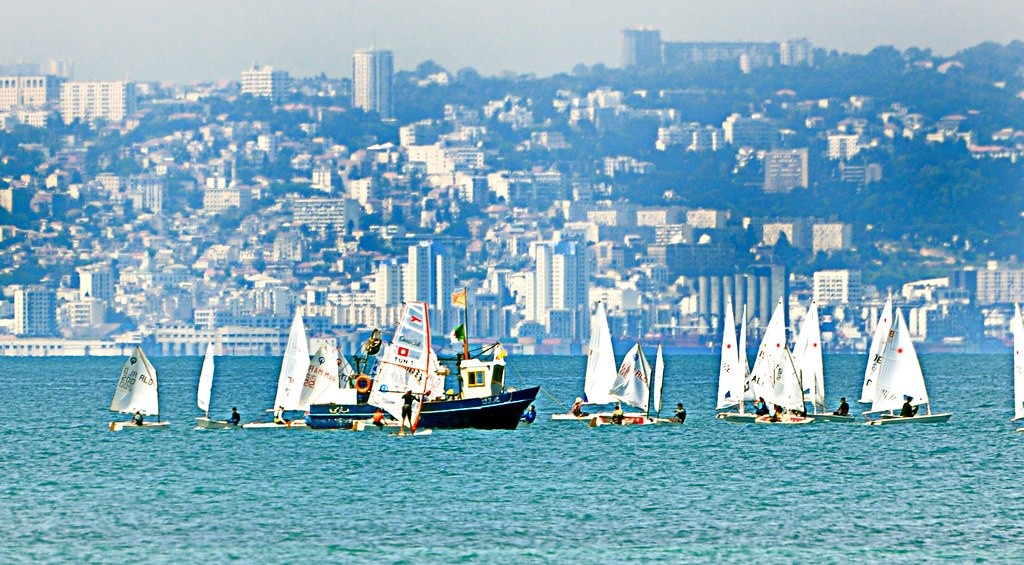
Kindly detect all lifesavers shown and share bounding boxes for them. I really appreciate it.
[355,374,370,393]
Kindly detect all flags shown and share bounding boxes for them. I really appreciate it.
[450,324,464,344]
[451,290,465,307]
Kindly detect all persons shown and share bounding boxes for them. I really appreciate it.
[769,403,782,422]
[796,406,806,416]
[274,406,290,424]
[521,405,536,424]
[754,397,767,415]
[132,410,143,426]
[422,390,432,401]
[228,407,240,426]
[611,406,623,425]
[912,405,918,416]
[900,396,914,417]
[670,403,686,423]
[572,397,589,417]
[372,409,388,431]
[833,397,849,416]
[398,389,420,434]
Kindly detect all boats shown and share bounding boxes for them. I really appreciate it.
[353,419,406,430]
[306,286,540,429]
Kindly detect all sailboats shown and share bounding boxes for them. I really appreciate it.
[551,298,685,426]
[1011,302,1024,433]
[196,342,234,429]
[716,292,855,425]
[366,297,433,437]
[859,289,952,426]
[109,345,170,430]
[241,305,311,428]
